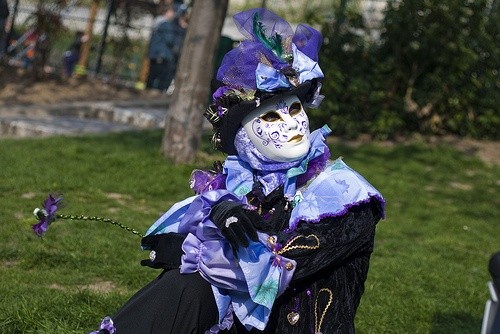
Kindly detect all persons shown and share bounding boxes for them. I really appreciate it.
[56,31,86,78]
[158,14,191,95]
[17,34,53,82]
[94,7,384,334]
[139,7,178,98]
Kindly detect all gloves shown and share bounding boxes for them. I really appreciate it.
[138,229,185,269]
[211,197,266,253]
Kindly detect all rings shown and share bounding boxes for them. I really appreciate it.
[241,203,258,211]
[225,217,238,228]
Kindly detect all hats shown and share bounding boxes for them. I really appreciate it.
[209,7,323,152]
